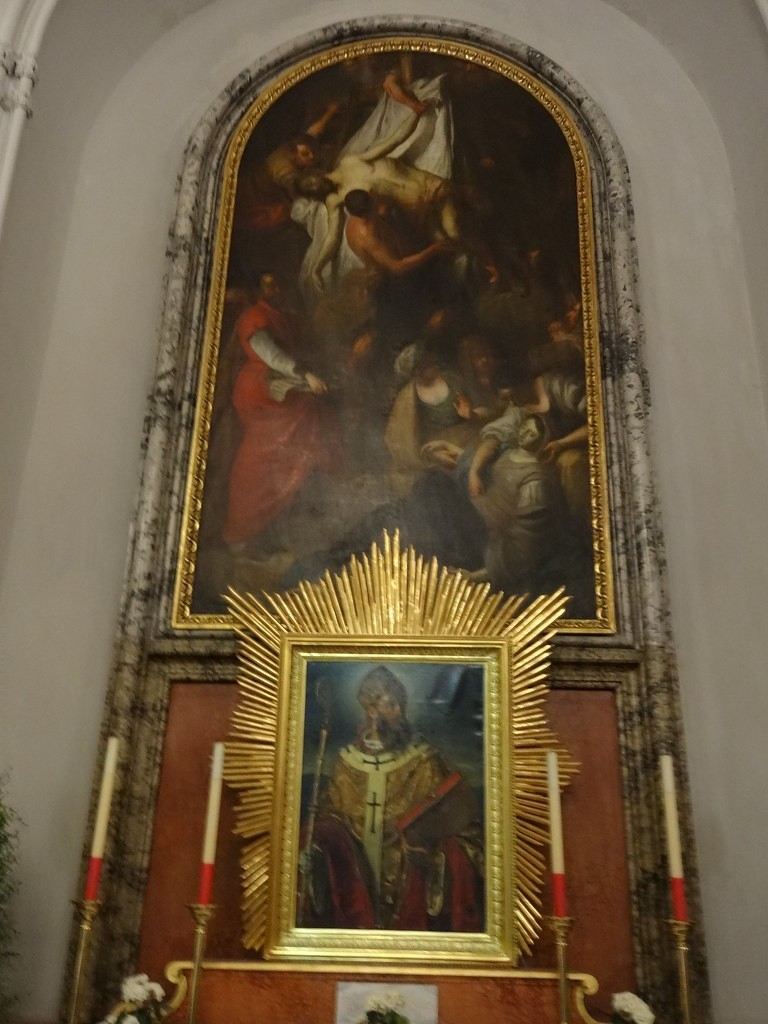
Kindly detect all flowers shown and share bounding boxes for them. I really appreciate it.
[123,975,171,1024]
[612,991,655,1024]
[354,986,415,1024]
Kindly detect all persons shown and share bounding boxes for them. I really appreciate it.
[298,665,486,938]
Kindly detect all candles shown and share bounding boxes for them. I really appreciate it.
[85,737,118,901]
[546,750,568,917]
[659,753,687,922]
[199,742,226,904]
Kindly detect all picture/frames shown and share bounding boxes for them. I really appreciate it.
[262,634,516,968]
